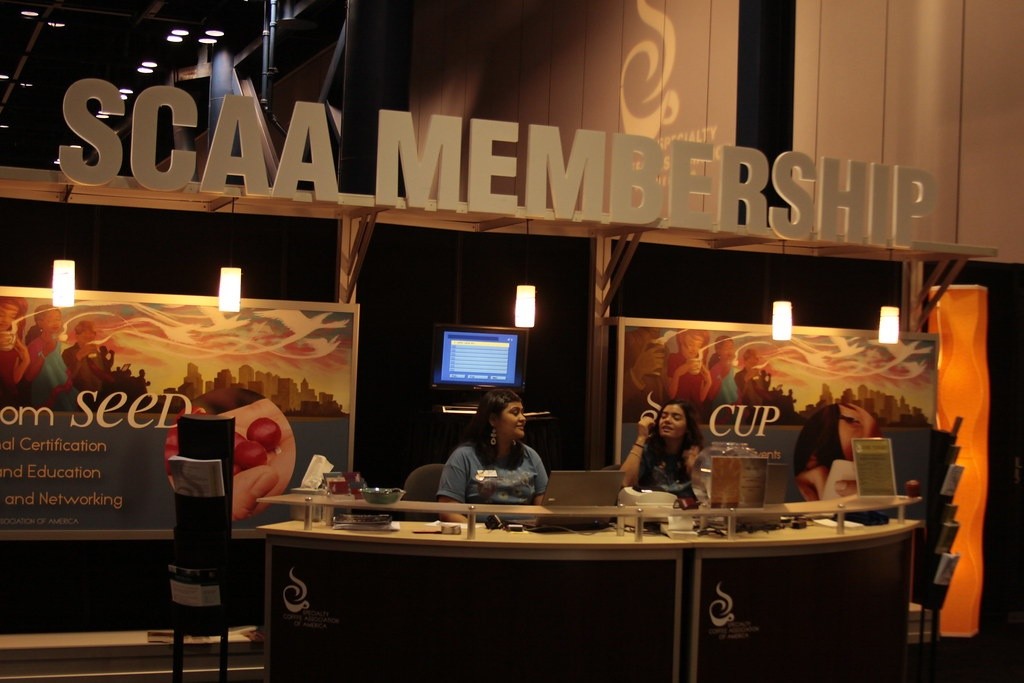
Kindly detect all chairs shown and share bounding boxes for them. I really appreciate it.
[402,462,445,522]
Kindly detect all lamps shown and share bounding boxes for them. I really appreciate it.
[769,240,793,343]
[878,249,901,346]
[514,220,536,330]
[217,198,242,315]
[51,189,77,310]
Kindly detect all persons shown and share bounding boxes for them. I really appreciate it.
[215,398,296,521]
[619,399,704,499]
[436,388,549,522]
[796,403,881,501]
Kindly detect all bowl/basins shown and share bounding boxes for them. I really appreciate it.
[359,487,406,504]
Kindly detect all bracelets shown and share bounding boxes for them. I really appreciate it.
[630,450,641,458]
[634,443,644,448]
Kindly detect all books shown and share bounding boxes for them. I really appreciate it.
[168,454,225,496]
[934,416,962,585]
[168,564,221,607]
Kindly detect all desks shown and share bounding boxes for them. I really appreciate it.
[254,490,925,683]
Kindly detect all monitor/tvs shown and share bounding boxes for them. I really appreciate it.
[430,324,530,394]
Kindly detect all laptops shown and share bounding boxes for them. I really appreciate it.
[761,463,791,526]
[506,469,626,532]
